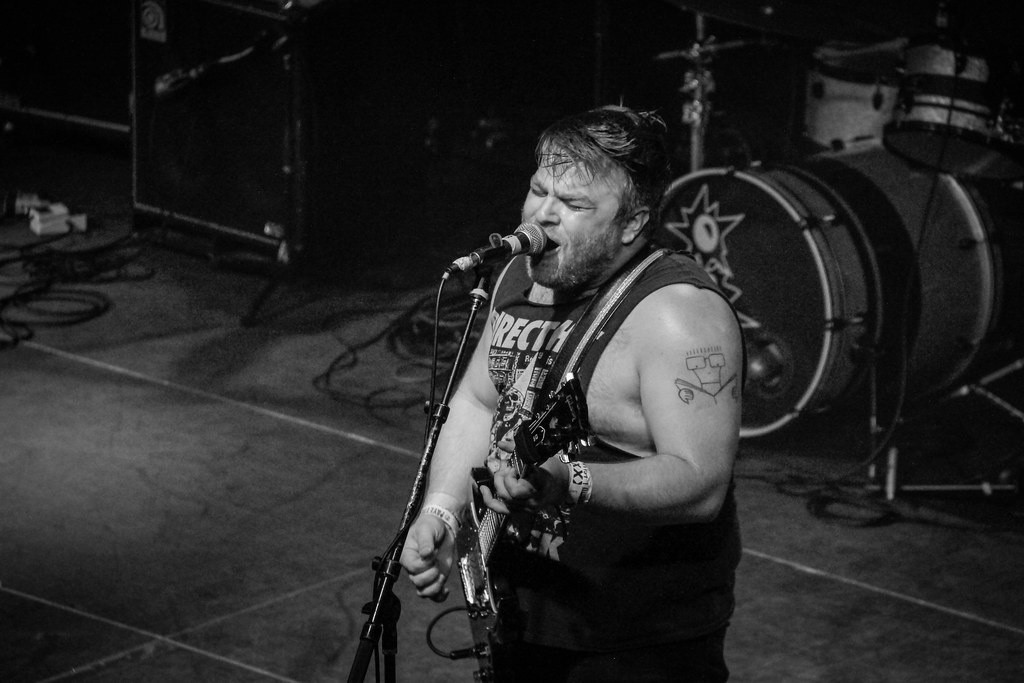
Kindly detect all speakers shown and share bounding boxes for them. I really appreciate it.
[127,0,303,261]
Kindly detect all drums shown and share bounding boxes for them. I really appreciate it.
[656,144,1004,442]
[801,40,905,148]
[883,40,1024,183]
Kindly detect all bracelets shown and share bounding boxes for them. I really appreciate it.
[421,507,459,537]
[568,461,593,503]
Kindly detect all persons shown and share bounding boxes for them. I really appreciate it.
[399,105,747,683]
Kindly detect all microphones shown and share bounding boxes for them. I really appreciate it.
[154,61,208,103]
[445,221,546,278]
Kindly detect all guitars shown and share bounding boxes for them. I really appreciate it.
[455,372,596,683]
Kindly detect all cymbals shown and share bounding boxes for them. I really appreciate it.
[670,0,915,40]
